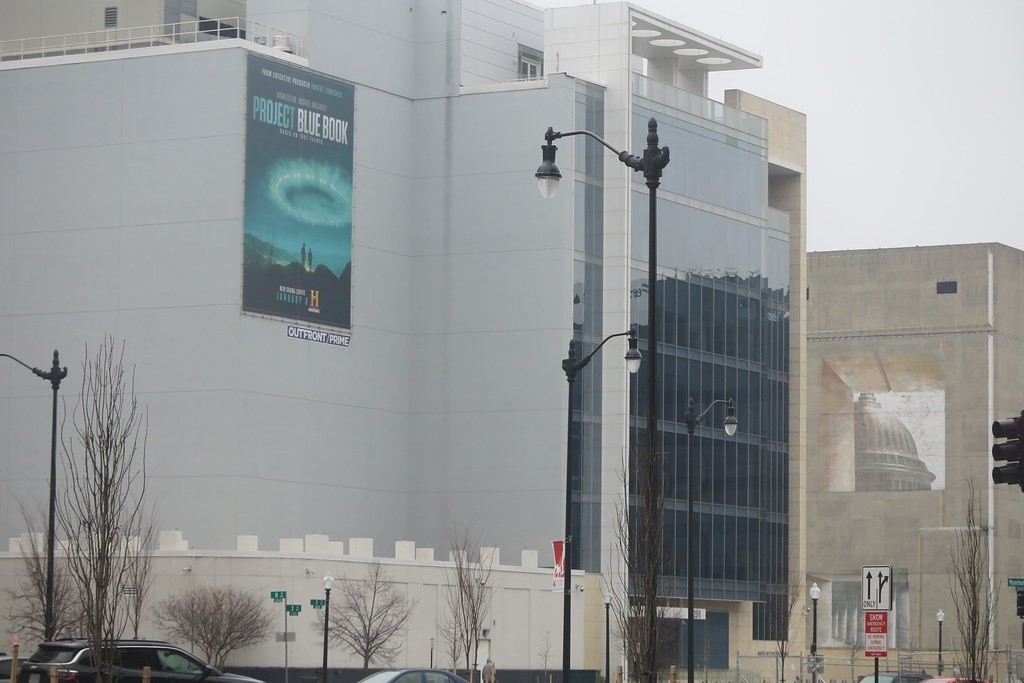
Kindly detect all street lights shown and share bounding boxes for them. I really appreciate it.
[561,325,642,683]
[532,115,672,683]
[602,588,613,683]
[322,575,334,683]
[684,396,739,683]
[935,608,946,678]
[809,582,821,683]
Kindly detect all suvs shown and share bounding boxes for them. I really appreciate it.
[17,637,266,683]
[857,669,933,683]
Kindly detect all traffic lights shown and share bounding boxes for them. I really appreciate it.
[990,408,1024,493]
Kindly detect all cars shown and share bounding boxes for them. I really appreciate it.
[0,654,31,683]
[356,666,468,683]
